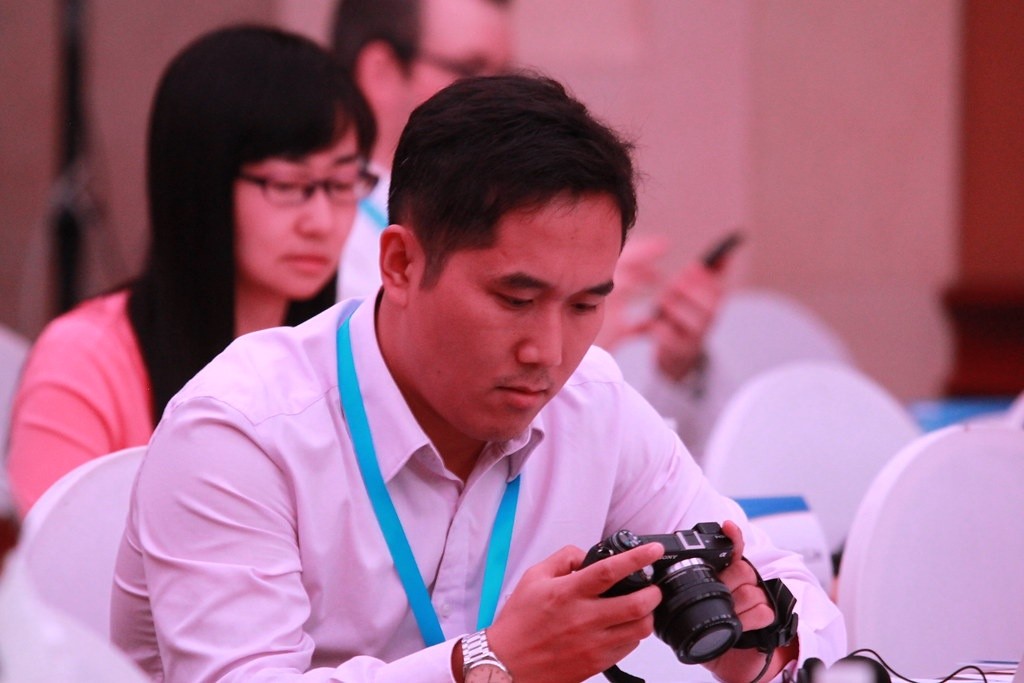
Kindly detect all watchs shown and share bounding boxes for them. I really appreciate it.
[460,627,512,683]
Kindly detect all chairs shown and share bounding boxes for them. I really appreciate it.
[0,292,1024,683]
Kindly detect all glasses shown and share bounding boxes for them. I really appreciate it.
[237,161,382,207]
[407,43,524,87]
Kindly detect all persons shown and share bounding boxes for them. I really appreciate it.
[111,72,847,683]
[330,0,744,422]
[9,21,382,515]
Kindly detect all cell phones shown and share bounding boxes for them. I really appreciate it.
[651,233,744,321]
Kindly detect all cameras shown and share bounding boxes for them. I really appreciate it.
[581,522,743,665]
